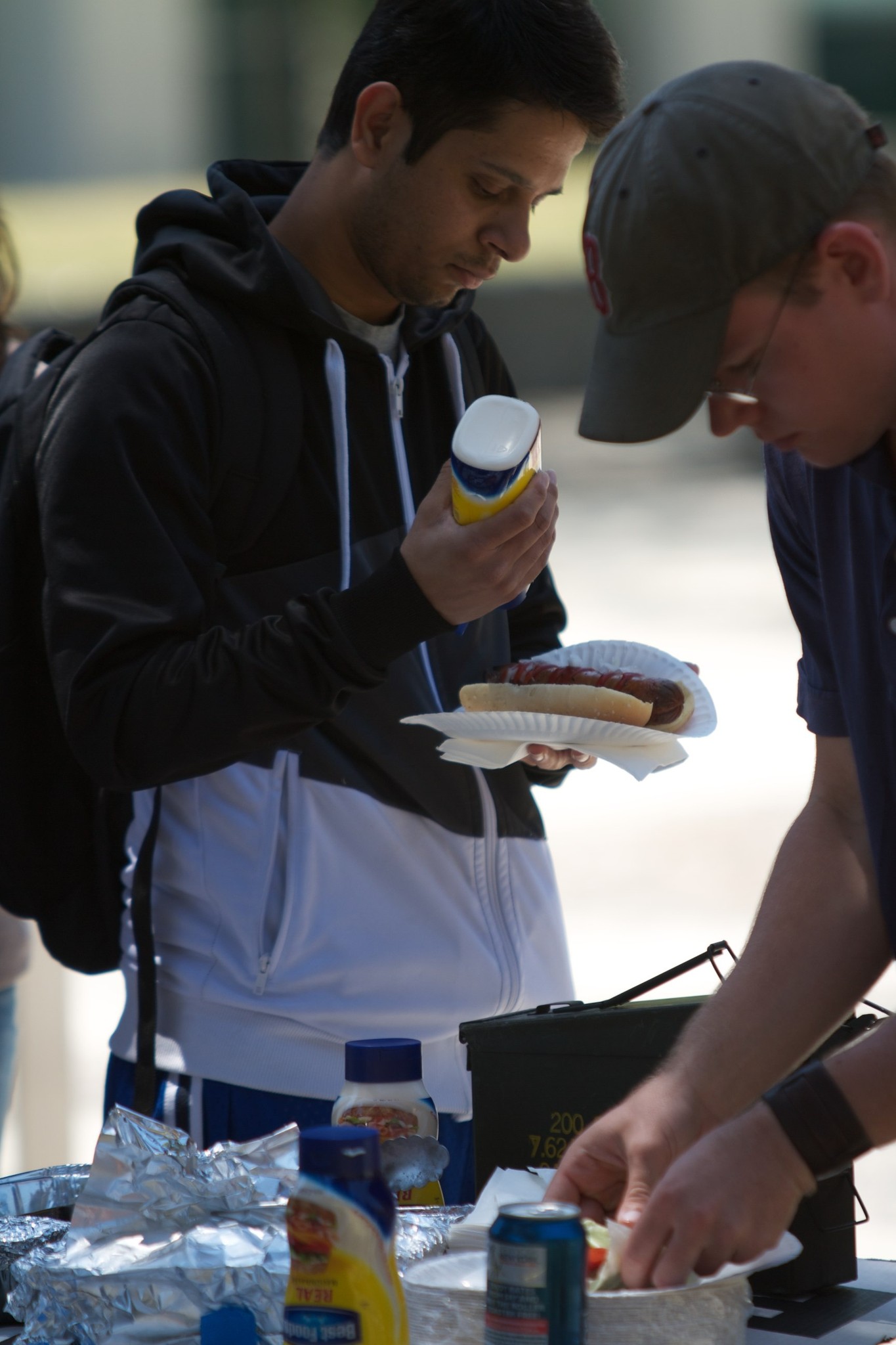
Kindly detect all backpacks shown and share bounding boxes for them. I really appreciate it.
[0,325,161,975]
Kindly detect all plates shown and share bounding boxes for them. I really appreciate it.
[460,1228,805,1299]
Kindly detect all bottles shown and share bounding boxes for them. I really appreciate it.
[281,1122,411,1344]
[332,1038,447,1206]
[448,393,543,616]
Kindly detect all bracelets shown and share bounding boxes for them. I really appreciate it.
[764,1063,872,1185]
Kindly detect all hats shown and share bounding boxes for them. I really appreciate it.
[575,60,887,447]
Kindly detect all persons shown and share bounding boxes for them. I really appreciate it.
[540,59,896,1290]
[39,0,625,1163]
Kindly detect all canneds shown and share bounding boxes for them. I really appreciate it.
[482,1203,586,1345]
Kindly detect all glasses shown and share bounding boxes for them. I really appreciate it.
[713,212,820,379]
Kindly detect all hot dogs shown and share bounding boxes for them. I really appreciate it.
[458,663,695,732]
